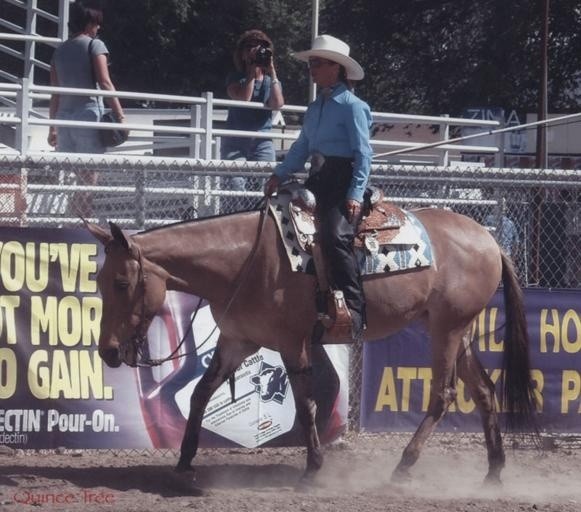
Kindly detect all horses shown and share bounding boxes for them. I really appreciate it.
[75,206,549,494]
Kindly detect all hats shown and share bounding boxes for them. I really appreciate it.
[288,34,364,80]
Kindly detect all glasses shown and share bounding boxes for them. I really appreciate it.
[308,59,330,68]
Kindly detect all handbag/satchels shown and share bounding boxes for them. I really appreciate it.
[100,111,127,147]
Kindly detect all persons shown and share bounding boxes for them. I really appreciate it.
[48,8,129,219]
[265,35,373,340]
[220,29,285,212]
[484,197,519,287]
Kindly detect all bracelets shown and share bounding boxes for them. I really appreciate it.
[115,115,124,121]
[271,79,278,84]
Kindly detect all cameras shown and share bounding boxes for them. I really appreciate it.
[256,45,269,58]
